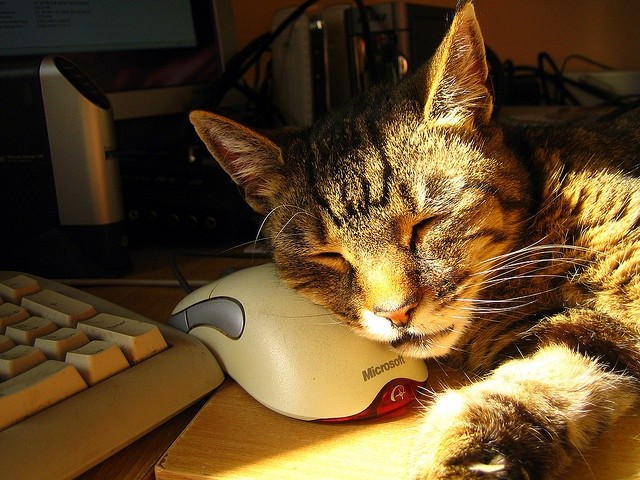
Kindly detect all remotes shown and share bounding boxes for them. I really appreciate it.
[170,263,429,424]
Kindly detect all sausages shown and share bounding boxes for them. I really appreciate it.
[187,0,640,480]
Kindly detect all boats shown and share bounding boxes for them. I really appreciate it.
[38,54,134,279]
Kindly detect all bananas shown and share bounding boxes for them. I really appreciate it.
[0,266,225,480]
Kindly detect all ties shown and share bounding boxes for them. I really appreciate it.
[0,1,248,162]
